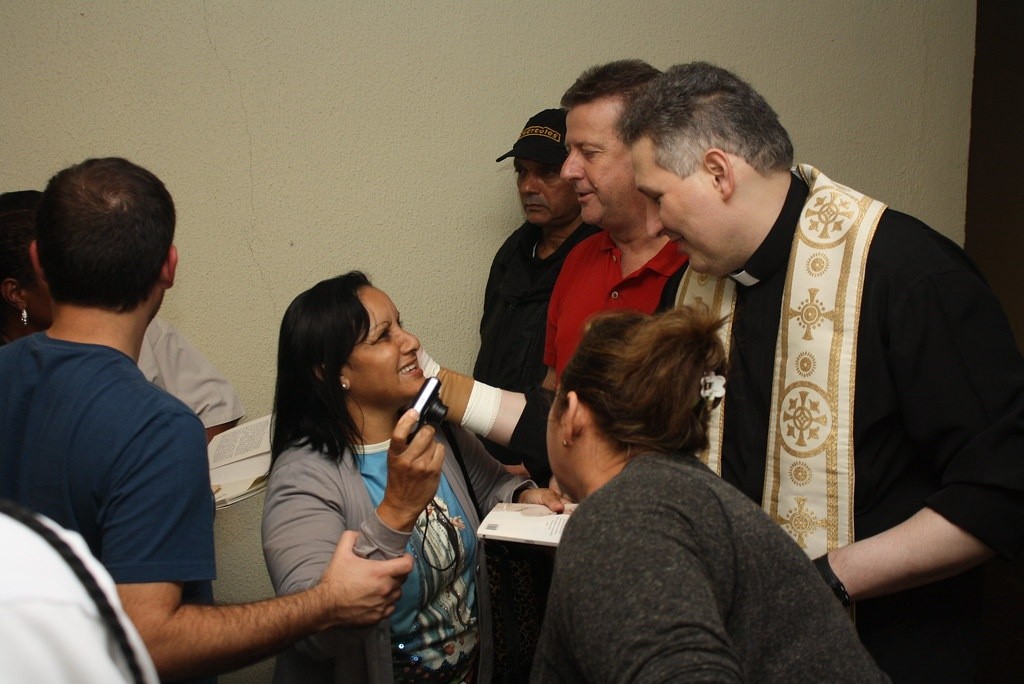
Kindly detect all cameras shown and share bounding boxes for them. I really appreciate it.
[403,377,450,444]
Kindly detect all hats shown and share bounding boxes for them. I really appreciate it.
[495,109,568,168]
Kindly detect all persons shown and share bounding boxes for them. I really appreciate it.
[530,310,892,684]
[262,269,571,684]
[413,62,1024,684]
[473,107,604,473]
[504,59,691,479]
[1,157,414,684]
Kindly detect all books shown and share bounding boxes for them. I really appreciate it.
[476,503,579,546]
[209,413,274,509]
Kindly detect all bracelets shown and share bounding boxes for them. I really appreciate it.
[812,553,850,606]
